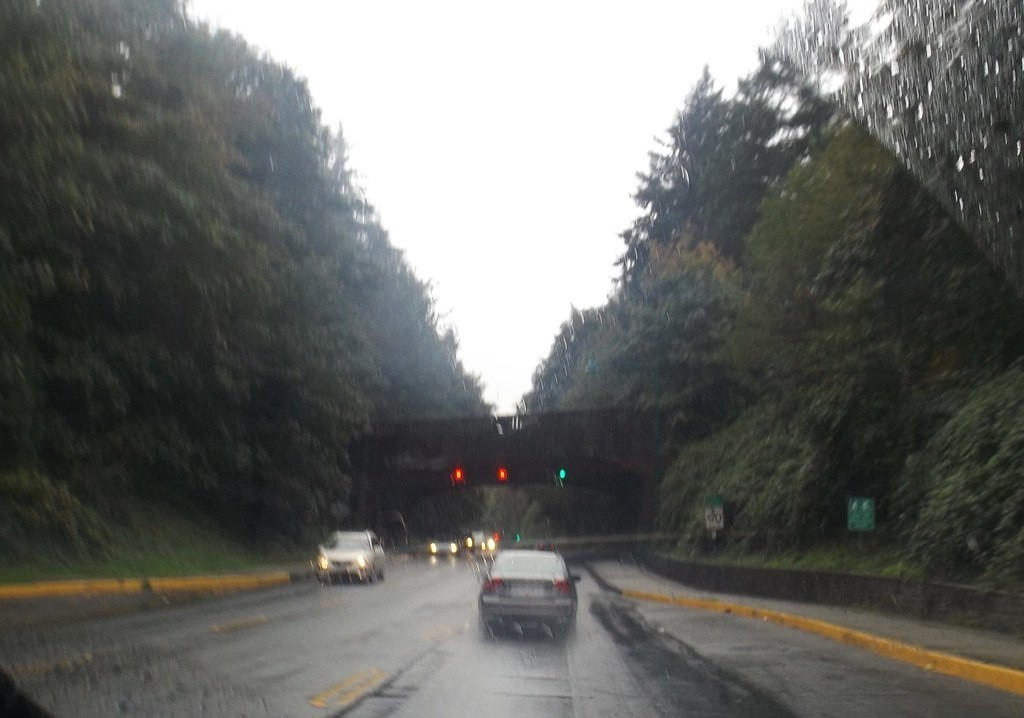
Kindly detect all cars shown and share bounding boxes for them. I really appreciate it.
[428,529,461,556]
[479,548,582,635]
[316,530,387,586]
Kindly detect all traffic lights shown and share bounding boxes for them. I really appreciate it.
[453,466,464,483]
[557,468,567,484]
[498,468,509,481]
